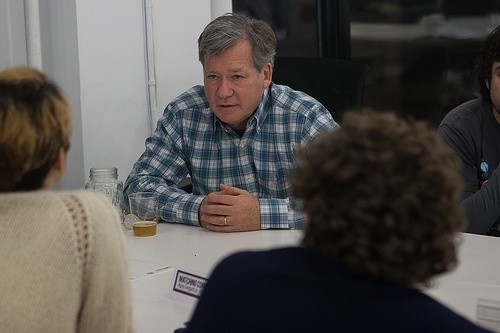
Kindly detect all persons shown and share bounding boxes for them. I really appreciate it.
[173,109,497,333]
[0,66,135,333]
[120,13,340,232]
[438,25,500,237]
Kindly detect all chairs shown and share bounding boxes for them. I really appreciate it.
[272,56,369,126]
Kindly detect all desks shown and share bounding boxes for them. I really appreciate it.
[124,222,500,333]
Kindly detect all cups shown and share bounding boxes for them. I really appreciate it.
[128,192,158,237]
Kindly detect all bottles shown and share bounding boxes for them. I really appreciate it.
[84,167,124,224]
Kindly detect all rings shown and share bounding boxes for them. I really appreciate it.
[224,216,228,226]
